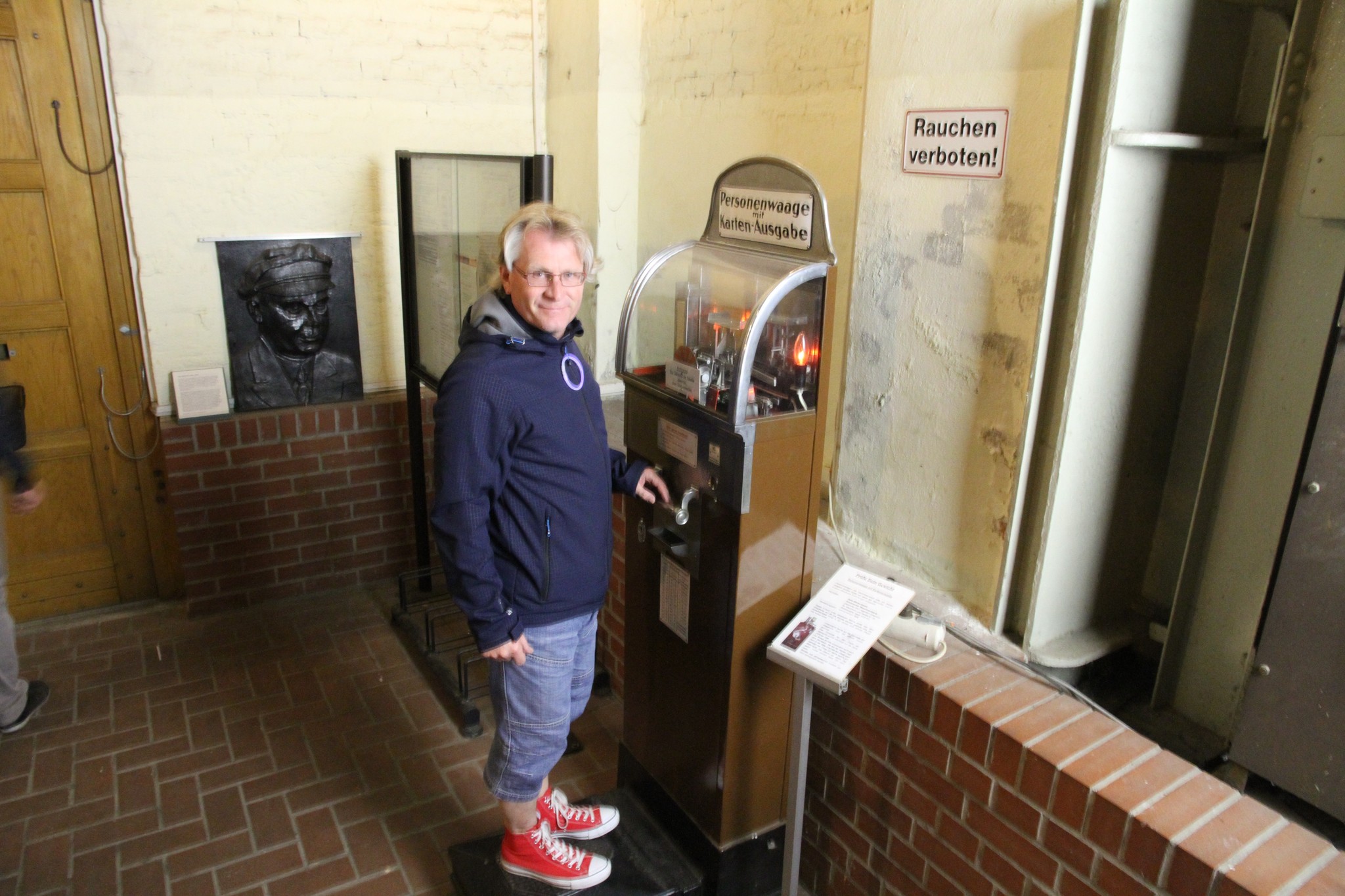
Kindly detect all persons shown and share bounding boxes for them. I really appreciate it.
[433,202,669,890]
[231,242,357,412]
[0,421,51,735]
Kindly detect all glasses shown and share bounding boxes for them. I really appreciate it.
[512,263,588,287]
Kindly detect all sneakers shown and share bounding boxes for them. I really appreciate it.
[499,809,612,890]
[536,784,621,840]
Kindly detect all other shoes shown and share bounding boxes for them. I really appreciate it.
[0,679,52,733]
